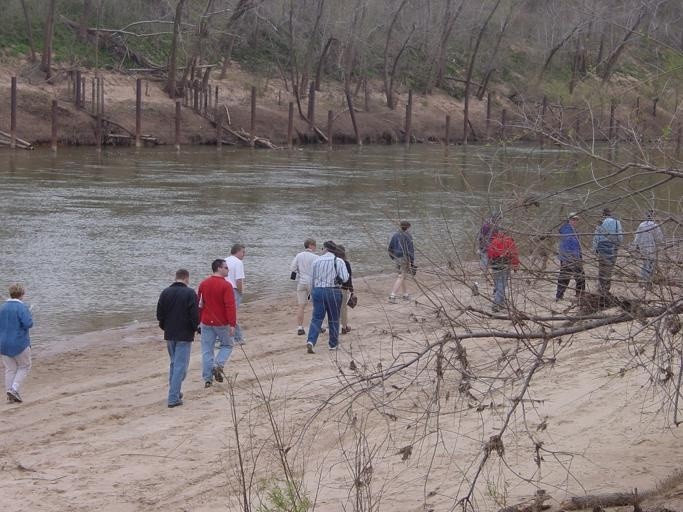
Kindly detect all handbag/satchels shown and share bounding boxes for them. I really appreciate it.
[347,294,358,307]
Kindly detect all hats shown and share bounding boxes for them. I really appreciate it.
[569,211,579,218]
[326,241,337,253]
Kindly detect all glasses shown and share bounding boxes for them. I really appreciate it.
[572,219,579,221]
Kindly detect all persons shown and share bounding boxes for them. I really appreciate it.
[474,212,497,289]
[0,283,34,402]
[222,243,245,345]
[306,241,349,354]
[630,210,663,290]
[156,269,199,407]
[335,245,353,334]
[289,237,325,335]
[387,221,413,303]
[488,221,519,313]
[196,259,236,387]
[589,208,622,292]
[555,211,585,301]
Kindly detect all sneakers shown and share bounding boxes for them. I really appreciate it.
[6,388,23,403]
[204,379,215,387]
[167,399,184,408]
[400,293,411,300]
[388,295,398,304]
[298,326,352,354]
[212,366,224,383]
[178,392,184,398]
